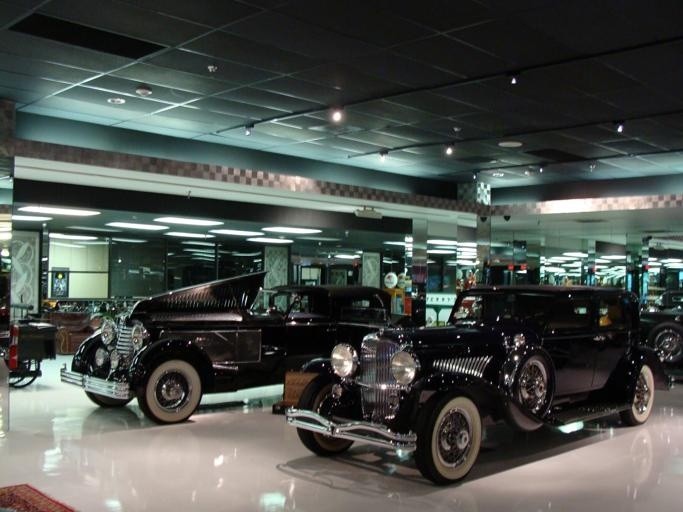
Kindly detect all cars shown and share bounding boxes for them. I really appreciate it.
[287,283,655,484]
[640,288,683,365]
[60,271,422,423]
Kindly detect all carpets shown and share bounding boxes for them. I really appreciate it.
[0,484,77,512]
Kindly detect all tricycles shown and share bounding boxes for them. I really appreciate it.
[0,318,58,388]
